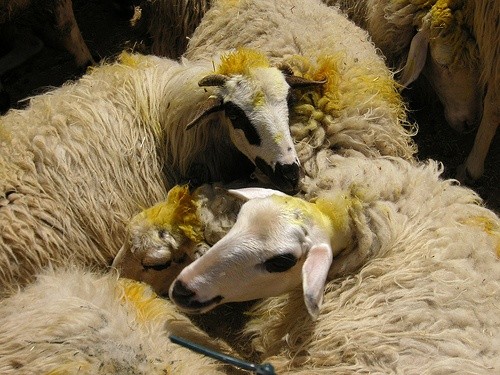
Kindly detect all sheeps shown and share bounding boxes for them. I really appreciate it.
[1,1,500,374]
